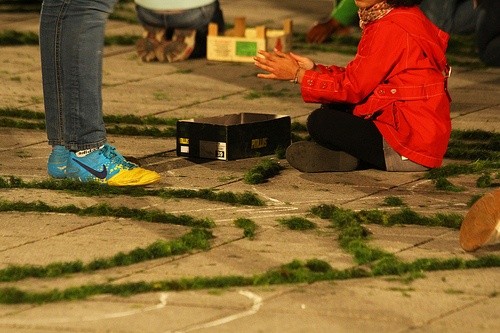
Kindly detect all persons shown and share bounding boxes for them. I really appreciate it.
[253,0,454,173]
[135,0,225,61]
[39,0,161,186]
[305,0,500,67]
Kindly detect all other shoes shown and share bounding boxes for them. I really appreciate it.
[459,187,500,253]
[135,22,167,62]
[286,139,357,172]
[167,26,197,62]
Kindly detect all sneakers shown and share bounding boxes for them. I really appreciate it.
[48,144,69,178]
[65,144,160,186]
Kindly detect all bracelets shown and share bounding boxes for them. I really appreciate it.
[290,67,301,86]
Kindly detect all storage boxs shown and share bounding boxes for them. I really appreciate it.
[206,28,293,64]
[177,112,292,162]
